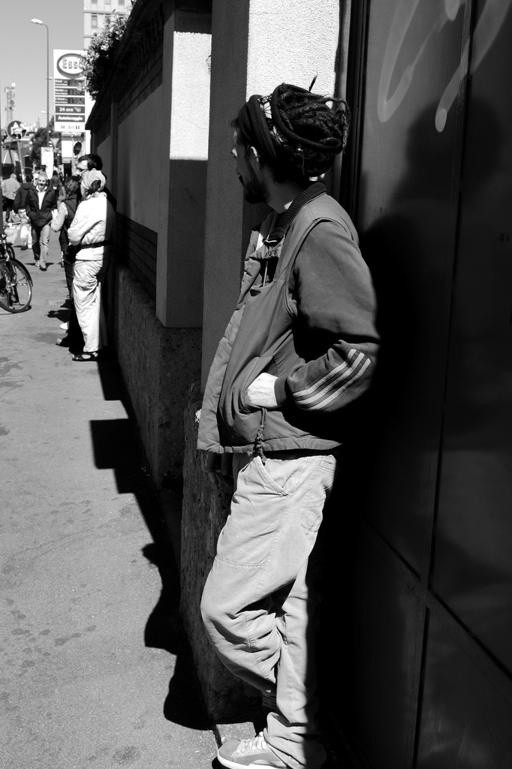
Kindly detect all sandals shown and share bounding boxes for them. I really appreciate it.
[72,351,99,361]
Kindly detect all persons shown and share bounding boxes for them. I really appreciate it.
[0,151,121,362]
[189,80,388,767]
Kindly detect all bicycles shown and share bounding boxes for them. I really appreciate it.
[0,229,34,311]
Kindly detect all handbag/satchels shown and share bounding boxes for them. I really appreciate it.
[12,224,32,251]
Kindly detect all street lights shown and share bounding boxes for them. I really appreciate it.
[41,110,53,117]
[5,82,17,122]
[31,17,51,146]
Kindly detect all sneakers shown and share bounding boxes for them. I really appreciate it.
[216,693,328,769]
[56,321,77,346]
